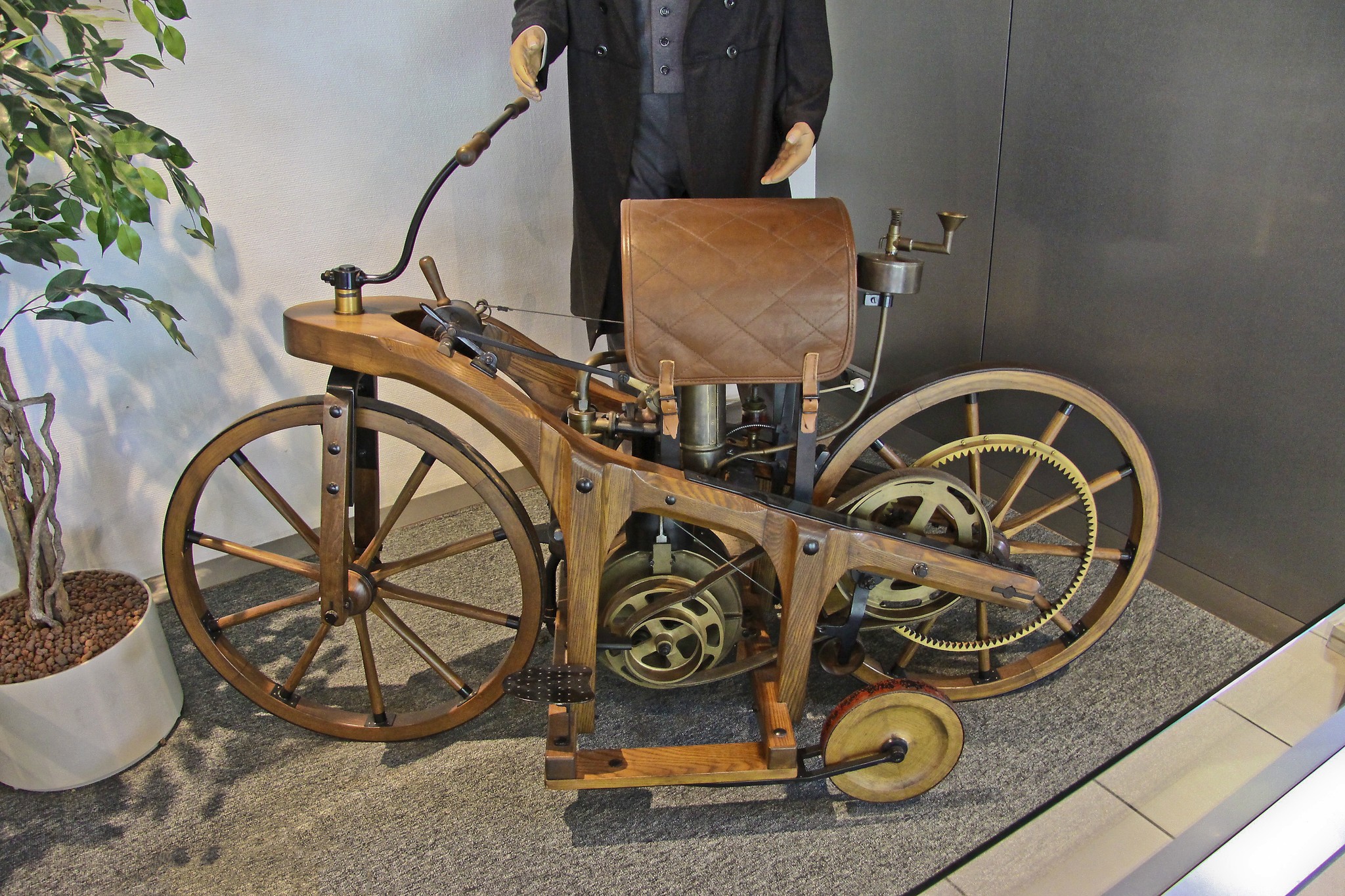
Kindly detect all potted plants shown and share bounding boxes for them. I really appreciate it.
[0,3,201,795]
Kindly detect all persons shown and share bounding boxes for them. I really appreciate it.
[508,0,834,548]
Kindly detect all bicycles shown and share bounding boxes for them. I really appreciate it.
[160,91,1165,806]
